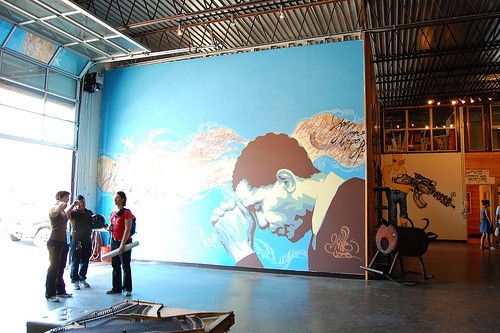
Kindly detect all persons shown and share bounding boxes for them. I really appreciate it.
[480,199,500,250]
[45,191,73,301]
[105,191,135,296]
[66,194,94,289]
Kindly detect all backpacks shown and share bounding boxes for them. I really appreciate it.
[114,208,136,236]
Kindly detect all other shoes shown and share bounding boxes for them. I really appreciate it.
[46,296,59,302]
[79,280,90,287]
[57,293,71,297]
[125,290,131,296]
[71,282,80,290]
[105,289,122,294]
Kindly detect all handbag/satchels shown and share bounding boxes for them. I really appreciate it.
[494,227,499,236]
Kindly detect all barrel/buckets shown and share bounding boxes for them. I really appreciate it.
[101,246,112,262]
